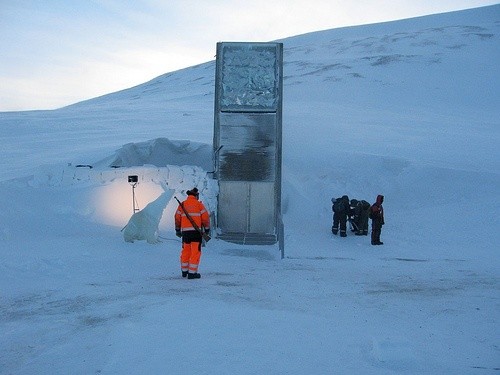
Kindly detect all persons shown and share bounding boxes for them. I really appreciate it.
[349,199,369,236]
[331,195,352,237]
[175,187,211,280]
[371,195,385,245]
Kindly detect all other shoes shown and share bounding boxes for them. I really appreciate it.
[182,271,188,277]
[188,273,201,279]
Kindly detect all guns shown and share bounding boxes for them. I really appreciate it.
[175,196,212,243]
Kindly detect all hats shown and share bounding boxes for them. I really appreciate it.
[186,187,198,196]
